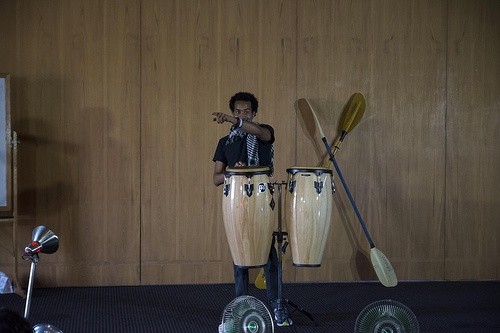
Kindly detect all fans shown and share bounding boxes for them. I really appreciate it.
[216,296,276,333]
[354,299,420,333]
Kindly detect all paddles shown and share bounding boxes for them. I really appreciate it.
[304,96,398,288]
[254,92,366,290]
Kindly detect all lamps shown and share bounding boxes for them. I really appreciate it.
[20,223,60,320]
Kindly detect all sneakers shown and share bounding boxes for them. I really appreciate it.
[273,306,292,326]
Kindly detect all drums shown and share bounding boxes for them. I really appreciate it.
[222,165,275,269]
[284,166,333,268]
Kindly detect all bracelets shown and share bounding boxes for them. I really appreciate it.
[239,118,243,128]
[234,117,240,128]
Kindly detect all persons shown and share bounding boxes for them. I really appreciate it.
[0,307,34,333]
[211,91,293,327]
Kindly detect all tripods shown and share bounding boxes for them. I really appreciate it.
[265,182,319,327]
